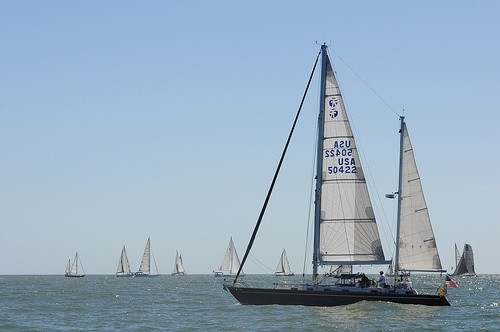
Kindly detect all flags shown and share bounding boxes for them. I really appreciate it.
[445,275,459,288]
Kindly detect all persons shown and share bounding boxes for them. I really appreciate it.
[376,271,385,287]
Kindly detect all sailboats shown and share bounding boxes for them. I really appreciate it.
[213,236,245,277]
[363,115,451,306]
[222,39,392,308]
[275,248,295,276]
[133,235,161,278]
[64,252,86,277]
[115,245,135,277]
[171,250,186,276]
[449,243,478,278]
